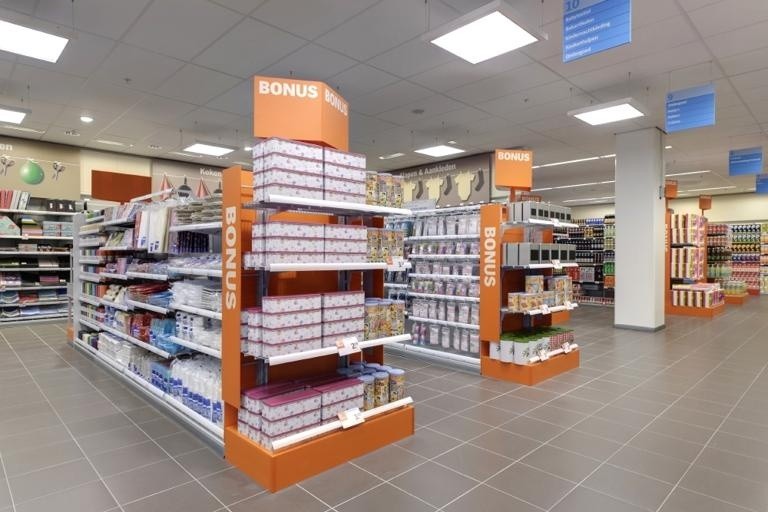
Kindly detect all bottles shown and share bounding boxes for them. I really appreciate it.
[567,217,604,263]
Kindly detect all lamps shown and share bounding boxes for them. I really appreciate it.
[420,0,548,68]
[0,0,69,65]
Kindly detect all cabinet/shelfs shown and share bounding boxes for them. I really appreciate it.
[66,181,236,447]
[241,156,409,447]
[671,214,768,306]
[502,200,578,366]
[2,190,74,326]
[383,208,478,373]
[572,215,614,306]
